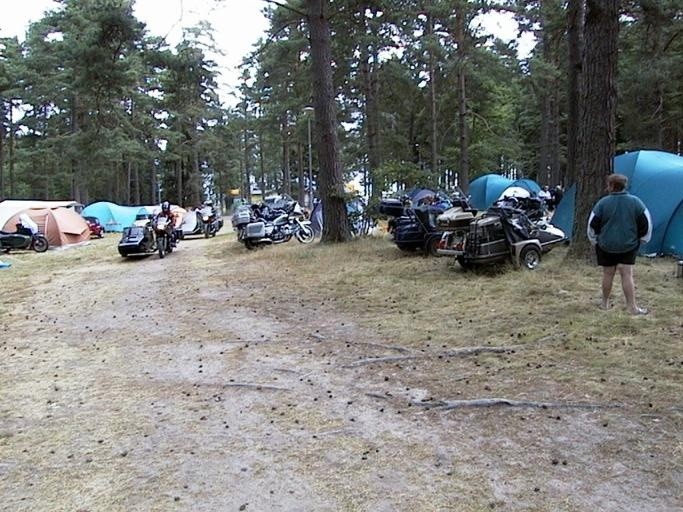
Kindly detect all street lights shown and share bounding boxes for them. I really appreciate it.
[302,107,315,198]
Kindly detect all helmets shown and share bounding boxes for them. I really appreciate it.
[162,201,170,213]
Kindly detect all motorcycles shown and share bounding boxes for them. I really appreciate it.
[176,204,222,240]
[387,192,568,273]
[0,213,48,254]
[233,199,314,251]
[118,208,176,259]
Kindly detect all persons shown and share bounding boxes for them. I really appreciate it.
[542,184,565,212]
[586,173,653,315]
[400,195,439,216]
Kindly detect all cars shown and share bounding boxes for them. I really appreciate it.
[378,187,435,218]
[258,195,282,204]
[80,216,104,238]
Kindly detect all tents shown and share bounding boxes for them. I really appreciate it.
[195,199,216,220]
[549,150,683,261]
[468,173,542,212]
[309,196,370,239]
[0,200,197,249]
[407,188,436,204]
